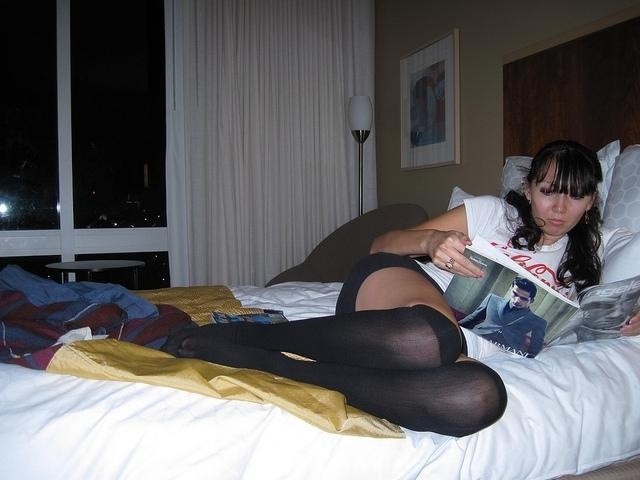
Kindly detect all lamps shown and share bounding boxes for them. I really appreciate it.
[346,94,374,217]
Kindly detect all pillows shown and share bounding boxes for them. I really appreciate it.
[585,227,640,291]
[498,139,620,223]
[600,145,639,230]
[447,186,477,214]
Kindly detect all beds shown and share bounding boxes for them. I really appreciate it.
[2,13,640,478]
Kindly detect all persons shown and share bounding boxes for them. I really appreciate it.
[457,277,547,358]
[411,76,446,146]
[162,139,640,437]
[575,280,640,340]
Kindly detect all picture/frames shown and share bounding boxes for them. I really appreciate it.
[396,27,461,173]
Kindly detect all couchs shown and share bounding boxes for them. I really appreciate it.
[265,202,430,289]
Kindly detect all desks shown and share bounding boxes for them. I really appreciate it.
[45,259,145,292]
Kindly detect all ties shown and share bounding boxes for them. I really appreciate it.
[511,308,518,327]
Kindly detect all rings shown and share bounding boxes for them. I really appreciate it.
[445,258,455,269]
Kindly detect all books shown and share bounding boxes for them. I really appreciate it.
[210,312,289,324]
[442,234,640,358]
